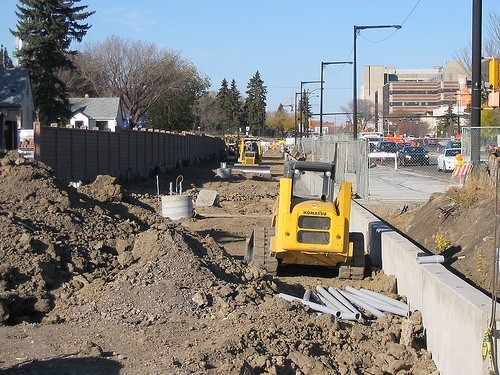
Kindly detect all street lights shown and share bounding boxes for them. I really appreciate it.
[294,92,310,145]
[352,24,404,140]
[299,81,326,140]
[319,61,354,135]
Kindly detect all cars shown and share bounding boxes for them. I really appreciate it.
[376,141,399,152]
[443,141,462,154]
[437,148,461,173]
[398,146,429,167]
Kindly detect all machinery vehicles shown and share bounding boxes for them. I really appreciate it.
[224,134,264,166]
[244,143,365,281]
